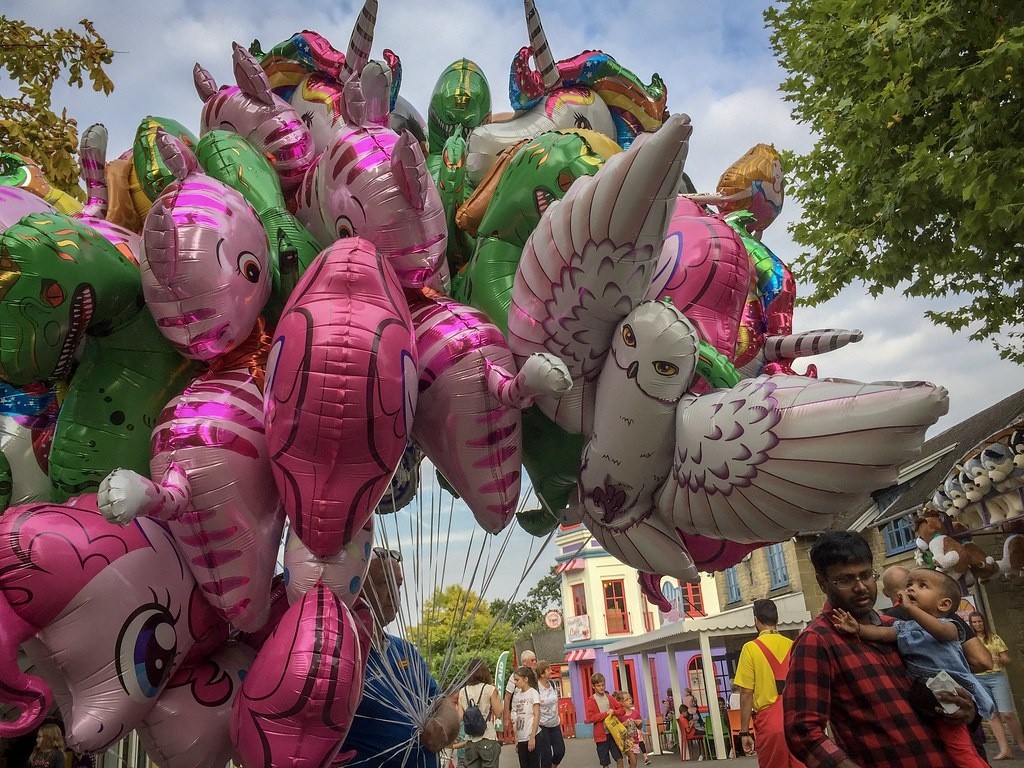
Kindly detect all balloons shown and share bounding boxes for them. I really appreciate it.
[0,0,949,768]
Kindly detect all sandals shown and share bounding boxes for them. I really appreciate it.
[993,753,1014,761]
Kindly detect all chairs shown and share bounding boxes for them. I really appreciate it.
[662,716,680,755]
[675,718,705,761]
[727,709,756,759]
[705,715,733,760]
[644,716,668,750]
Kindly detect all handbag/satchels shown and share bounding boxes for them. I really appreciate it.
[604,713,634,754]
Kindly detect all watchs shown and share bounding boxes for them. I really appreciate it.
[738,731,750,737]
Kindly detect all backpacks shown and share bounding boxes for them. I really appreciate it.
[463,682,492,735]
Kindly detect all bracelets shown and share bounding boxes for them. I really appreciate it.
[857,623,861,637]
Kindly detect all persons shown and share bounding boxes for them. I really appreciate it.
[339,531,1024,768]
[28,724,66,768]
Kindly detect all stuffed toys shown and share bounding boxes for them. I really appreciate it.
[913,428,1024,595]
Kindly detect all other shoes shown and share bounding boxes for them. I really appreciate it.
[729,748,734,759]
[698,755,703,761]
[645,759,651,765]
[628,758,630,765]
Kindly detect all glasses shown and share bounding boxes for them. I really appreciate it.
[372,547,403,563]
[825,568,880,589]
